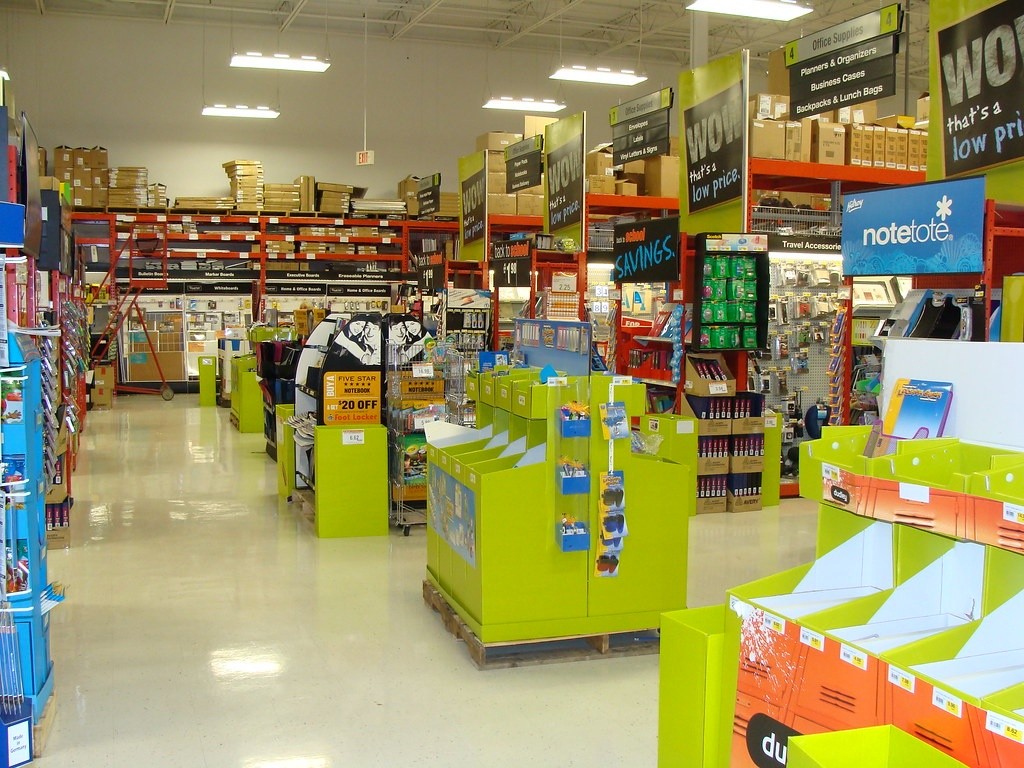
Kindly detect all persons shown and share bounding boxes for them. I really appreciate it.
[387,314,433,365]
[323,314,381,366]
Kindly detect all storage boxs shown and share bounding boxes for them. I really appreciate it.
[46,527,71,550]
[697,457,729,475]
[93,365,114,390]
[54,403,67,456]
[626,355,652,379]
[39,145,459,271]
[681,392,731,436]
[45,453,67,504]
[216,337,245,394]
[683,353,737,397]
[731,392,765,435]
[474,47,929,216]
[90,388,113,411]
[696,497,727,514]
[728,453,765,474]
[726,488,762,513]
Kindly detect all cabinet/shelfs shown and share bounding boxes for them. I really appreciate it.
[615,235,748,430]
[69,211,482,397]
[294,310,383,494]
[184,309,240,380]
[493,248,580,351]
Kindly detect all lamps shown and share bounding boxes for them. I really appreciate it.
[480,0,567,112]
[229,0,333,73]
[201,0,281,119]
[548,0,649,86]
[684,0,815,21]
